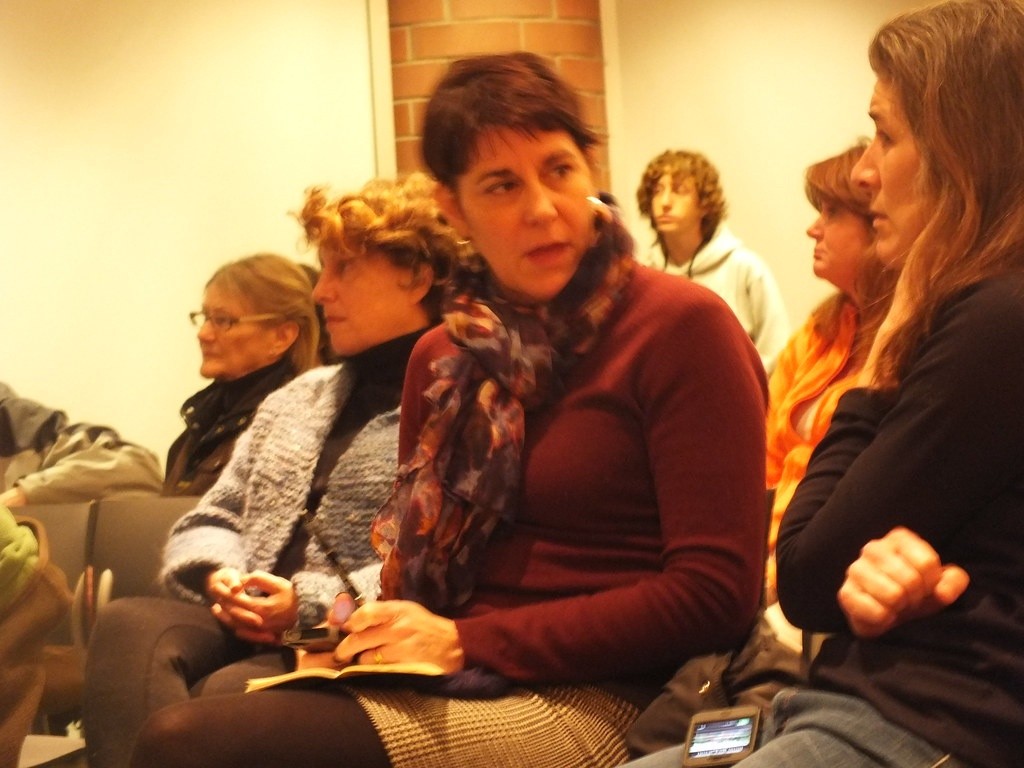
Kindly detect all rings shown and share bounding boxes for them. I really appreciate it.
[373,648,382,664]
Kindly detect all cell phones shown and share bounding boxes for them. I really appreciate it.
[281,626,346,653]
[683,705,760,768]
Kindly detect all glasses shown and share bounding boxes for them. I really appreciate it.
[191,311,285,330]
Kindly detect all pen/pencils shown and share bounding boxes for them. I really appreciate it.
[299,506,365,609]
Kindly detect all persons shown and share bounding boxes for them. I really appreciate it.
[154,255,337,500]
[632,152,787,374]
[765,134,899,608]
[127,51,770,767]
[83,172,476,767]
[617,2,1023,766]
[0,382,162,508]
